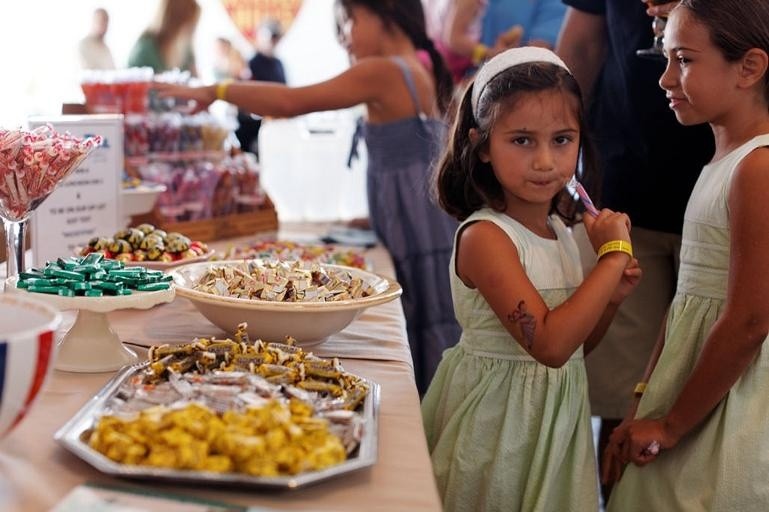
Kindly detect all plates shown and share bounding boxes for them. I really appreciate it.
[55,359,382,489]
[168,258,403,344]
[73,246,215,270]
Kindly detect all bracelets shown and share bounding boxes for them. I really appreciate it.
[632,382,647,396]
[596,240,633,263]
[216,78,234,99]
[471,45,486,67]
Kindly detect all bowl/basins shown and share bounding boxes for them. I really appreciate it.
[1,287,62,437]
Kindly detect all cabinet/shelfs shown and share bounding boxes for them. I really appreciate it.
[60,102,281,241]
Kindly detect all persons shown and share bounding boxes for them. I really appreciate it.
[601,0,769,512]
[151,0,454,398]
[554,0,715,236]
[213,19,286,152]
[420,46,641,512]
[127,0,201,78]
[75,8,114,71]
[444,0,569,70]
